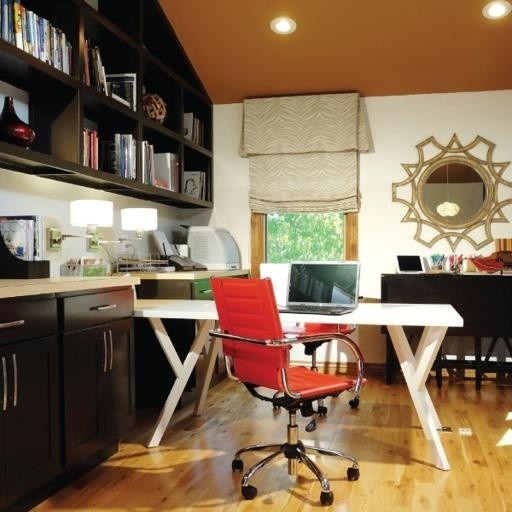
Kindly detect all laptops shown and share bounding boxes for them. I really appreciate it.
[277,261,359,316]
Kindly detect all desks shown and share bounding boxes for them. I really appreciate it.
[380,272,512,389]
[134,299,464,471]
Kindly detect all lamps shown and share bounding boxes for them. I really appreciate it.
[46,200,113,252]
[87,208,157,250]
[436,164,460,217]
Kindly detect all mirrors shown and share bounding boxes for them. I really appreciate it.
[392,133,512,254]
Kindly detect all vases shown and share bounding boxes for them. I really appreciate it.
[0,96,36,148]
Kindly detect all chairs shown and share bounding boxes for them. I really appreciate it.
[209,274,360,506]
[272,323,364,416]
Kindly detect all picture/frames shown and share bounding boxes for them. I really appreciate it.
[0,215,38,262]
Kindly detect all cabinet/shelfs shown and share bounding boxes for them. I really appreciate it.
[58,285,136,470]
[0,294,62,512]
[136,276,249,391]
[0,0,214,202]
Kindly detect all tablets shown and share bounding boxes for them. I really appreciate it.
[396,254,425,275]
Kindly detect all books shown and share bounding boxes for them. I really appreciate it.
[81,124,212,202]
[2,0,138,113]
[0,214,45,262]
[183,110,207,149]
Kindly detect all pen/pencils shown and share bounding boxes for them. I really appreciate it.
[431,254,444,271]
[449,254,463,273]
